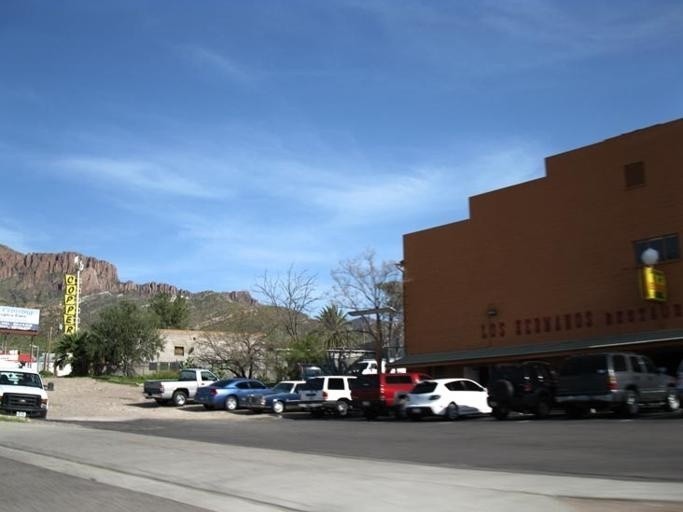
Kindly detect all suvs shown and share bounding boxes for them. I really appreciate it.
[0,367,54,418]
[556,351,682,416]
[487,362,561,417]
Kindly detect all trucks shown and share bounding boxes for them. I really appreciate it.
[350,357,406,376]
[294,362,322,380]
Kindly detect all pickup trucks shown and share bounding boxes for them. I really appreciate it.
[144,368,220,406]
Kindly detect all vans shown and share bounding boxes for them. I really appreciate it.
[300,375,359,416]
[351,372,434,421]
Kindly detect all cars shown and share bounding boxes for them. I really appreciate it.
[194,378,269,410]
[0,348,65,363]
[240,380,305,414]
[401,378,493,419]
